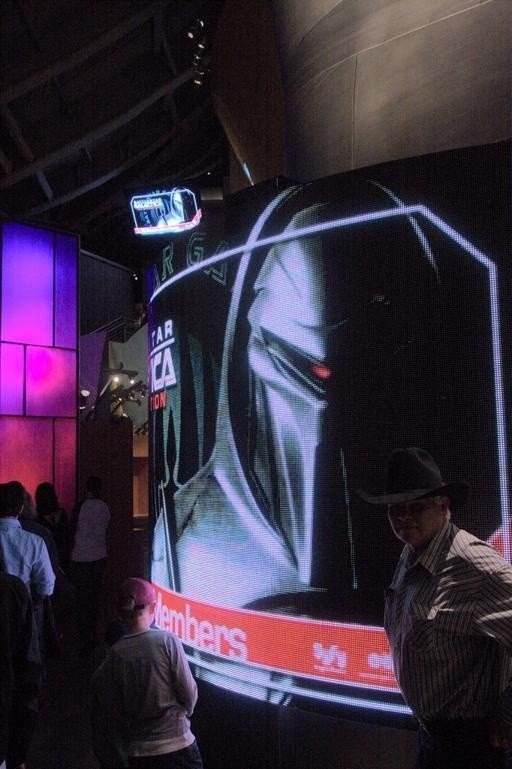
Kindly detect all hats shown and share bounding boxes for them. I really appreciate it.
[117,577,155,606]
[353,446,471,509]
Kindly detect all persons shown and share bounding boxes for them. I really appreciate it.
[348,446,512,768]
